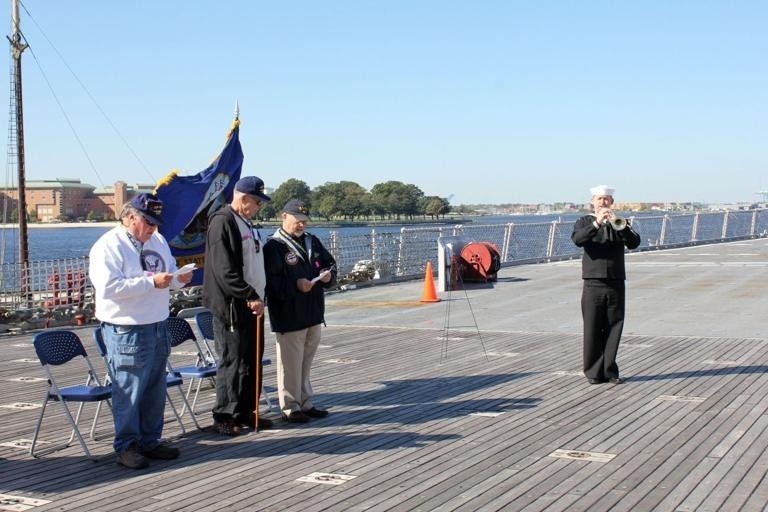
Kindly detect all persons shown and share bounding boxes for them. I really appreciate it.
[570,188,639,384]
[202,177,274,437]
[261,197,336,427]
[87,192,192,470]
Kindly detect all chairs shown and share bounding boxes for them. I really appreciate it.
[196,312,272,409]
[29,329,113,457]
[93,327,200,433]
[160,318,218,415]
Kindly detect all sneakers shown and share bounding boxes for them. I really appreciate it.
[282,410,310,423]
[302,407,328,419]
[213,421,237,436]
[116,445,149,470]
[144,444,180,460]
[245,414,272,430]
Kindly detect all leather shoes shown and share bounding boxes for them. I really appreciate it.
[609,377,622,384]
[589,378,600,384]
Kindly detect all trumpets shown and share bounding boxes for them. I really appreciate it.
[604,208,626,231]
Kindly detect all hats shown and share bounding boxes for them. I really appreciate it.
[131,192,166,226]
[235,176,270,202]
[590,186,616,196]
[283,200,309,222]
